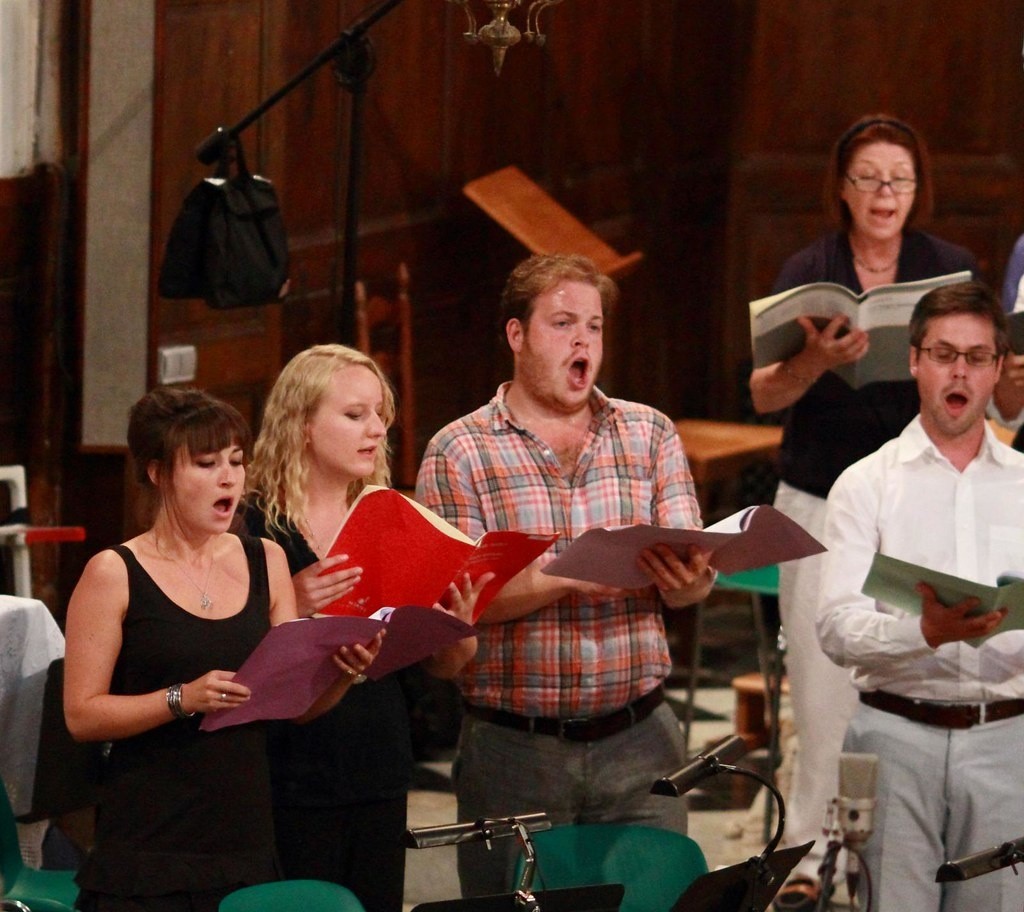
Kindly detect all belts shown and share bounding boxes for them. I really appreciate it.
[464,684,664,744]
[859,688,1024,729]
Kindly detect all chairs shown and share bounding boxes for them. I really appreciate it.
[219,880,367,912]
[513,822,710,912]
[0,776,81,912]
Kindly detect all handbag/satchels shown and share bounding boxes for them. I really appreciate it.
[156,126,292,310]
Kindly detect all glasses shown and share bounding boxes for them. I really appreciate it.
[841,171,918,194]
[918,346,998,368]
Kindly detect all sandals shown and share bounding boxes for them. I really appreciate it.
[775,872,819,911]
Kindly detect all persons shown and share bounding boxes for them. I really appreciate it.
[984,234,1024,452]
[63,385,386,912]
[227,344,494,912]
[736,113,981,912]
[414,253,716,898]
[813,282,1024,912]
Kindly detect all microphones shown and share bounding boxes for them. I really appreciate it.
[837,751,879,895]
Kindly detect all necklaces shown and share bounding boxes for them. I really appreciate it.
[153,538,214,609]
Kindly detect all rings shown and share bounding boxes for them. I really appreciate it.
[346,666,353,677]
[220,692,226,703]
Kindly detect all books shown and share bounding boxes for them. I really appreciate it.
[749,270,972,390]
[311,484,562,630]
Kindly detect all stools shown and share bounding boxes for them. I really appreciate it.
[644,423,782,756]
[729,673,790,807]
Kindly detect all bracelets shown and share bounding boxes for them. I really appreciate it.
[782,362,816,384]
[167,683,196,720]
